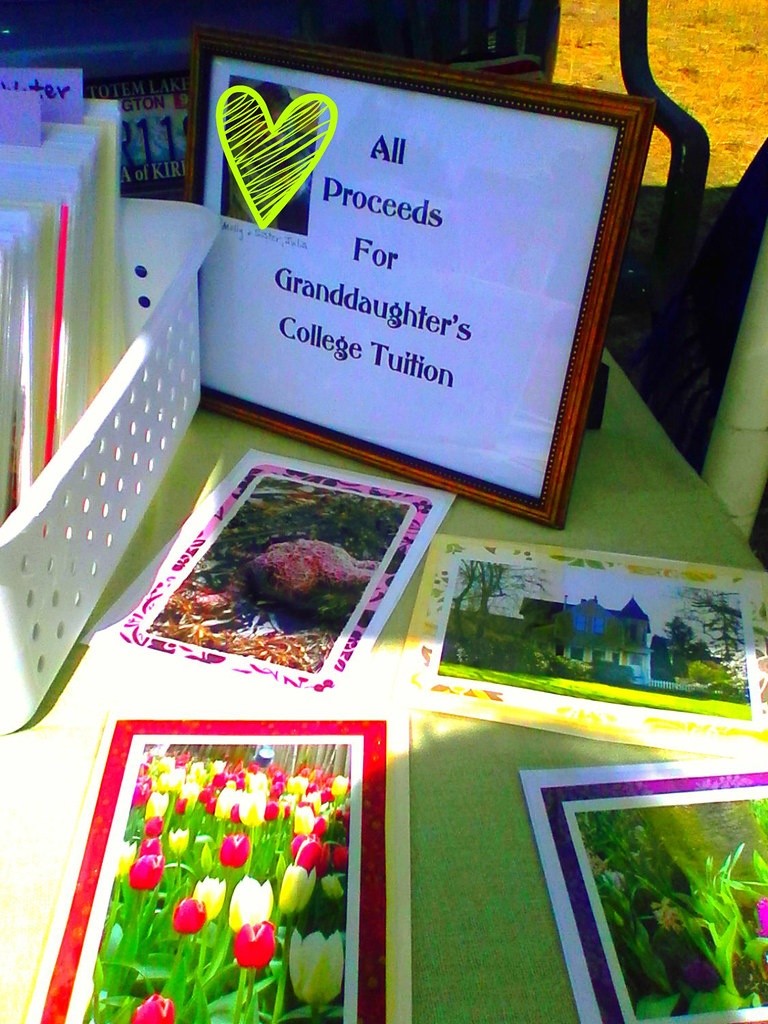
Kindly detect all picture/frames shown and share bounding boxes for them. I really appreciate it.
[182,26,660,527]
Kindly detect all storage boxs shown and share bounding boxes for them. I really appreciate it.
[1,196,223,737]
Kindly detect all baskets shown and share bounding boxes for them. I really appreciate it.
[0,195,224,726]
